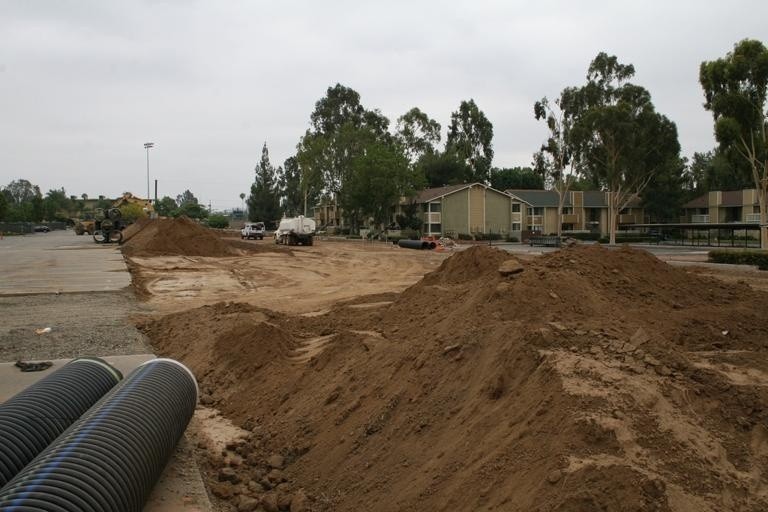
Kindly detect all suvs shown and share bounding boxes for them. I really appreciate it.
[239,221,266,241]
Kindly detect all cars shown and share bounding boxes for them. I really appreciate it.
[34,224,50,233]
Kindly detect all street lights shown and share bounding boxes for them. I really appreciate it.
[142,141,155,202]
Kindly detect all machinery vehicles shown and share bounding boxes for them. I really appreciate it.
[73,209,99,236]
[274,215,317,247]
